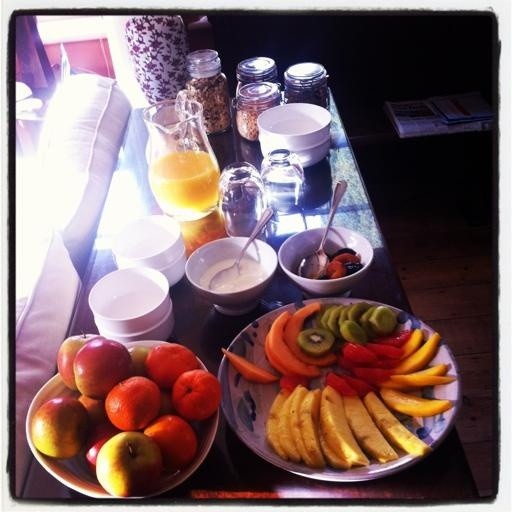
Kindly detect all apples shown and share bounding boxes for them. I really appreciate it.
[32,330,163,498]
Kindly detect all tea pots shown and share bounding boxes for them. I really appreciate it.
[142,99,222,227]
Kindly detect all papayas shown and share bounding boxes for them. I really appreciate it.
[223,304,336,384]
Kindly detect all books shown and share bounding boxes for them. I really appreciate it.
[384,89,494,138]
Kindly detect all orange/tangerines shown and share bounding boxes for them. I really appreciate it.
[105,344,222,469]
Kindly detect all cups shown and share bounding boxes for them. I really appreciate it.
[262,149,307,217]
[220,161,266,238]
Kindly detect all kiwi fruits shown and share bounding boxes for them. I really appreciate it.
[297,304,396,353]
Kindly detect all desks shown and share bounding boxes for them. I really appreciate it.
[18,80,486,506]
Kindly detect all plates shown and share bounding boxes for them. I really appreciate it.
[25,340,220,500]
[217,297,460,484]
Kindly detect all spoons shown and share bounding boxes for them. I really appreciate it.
[208,207,273,290]
[298,180,348,279]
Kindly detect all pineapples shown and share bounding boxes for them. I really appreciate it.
[265,329,457,470]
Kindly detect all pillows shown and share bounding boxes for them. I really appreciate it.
[15,234,82,503]
[33,70,130,281]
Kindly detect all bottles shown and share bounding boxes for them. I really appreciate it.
[186,46,330,141]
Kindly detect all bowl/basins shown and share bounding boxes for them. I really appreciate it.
[186,237,276,314]
[279,224,374,297]
[88,218,188,339]
[259,100,331,167]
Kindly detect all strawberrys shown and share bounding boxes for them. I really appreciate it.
[278,329,410,397]
